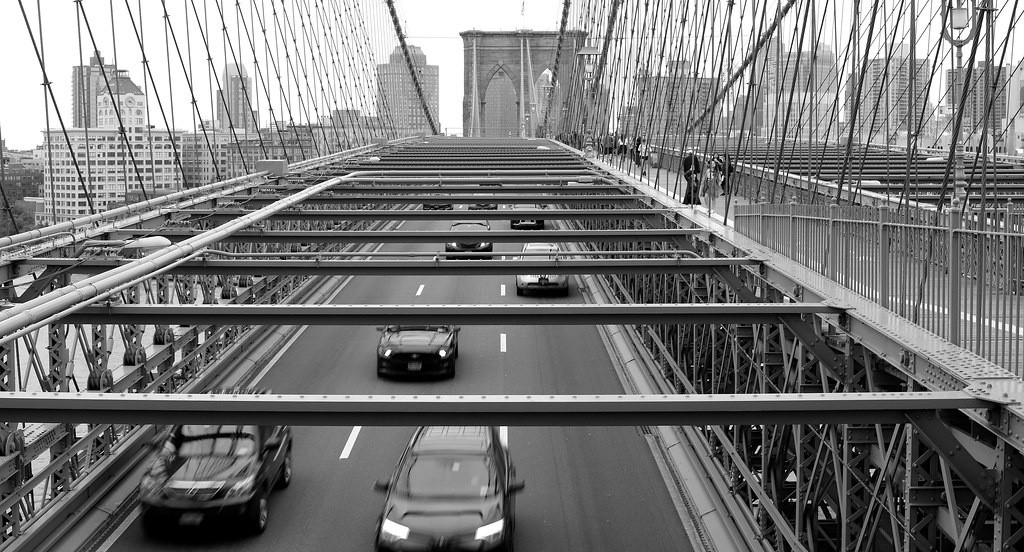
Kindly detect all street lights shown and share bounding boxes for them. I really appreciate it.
[940,0,977,215]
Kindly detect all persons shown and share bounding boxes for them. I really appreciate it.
[720,153,731,196]
[682,165,701,205]
[601,132,628,162]
[702,162,723,214]
[684,149,700,183]
[638,139,652,176]
[708,152,723,184]
[634,137,642,166]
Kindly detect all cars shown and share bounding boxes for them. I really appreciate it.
[479,174,501,187]
[466,203,499,209]
[375,322,463,383]
[422,199,454,210]
[507,203,549,230]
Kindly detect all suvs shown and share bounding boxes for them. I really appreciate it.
[446,218,494,260]
[373,423,526,552]
[515,242,570,295]
[138,389,295,539]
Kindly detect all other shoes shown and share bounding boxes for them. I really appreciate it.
[720,192,726,196]
[710,210,714,214]
[643,171,646,176]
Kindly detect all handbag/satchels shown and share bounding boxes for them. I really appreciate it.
[730,166,733,173]
